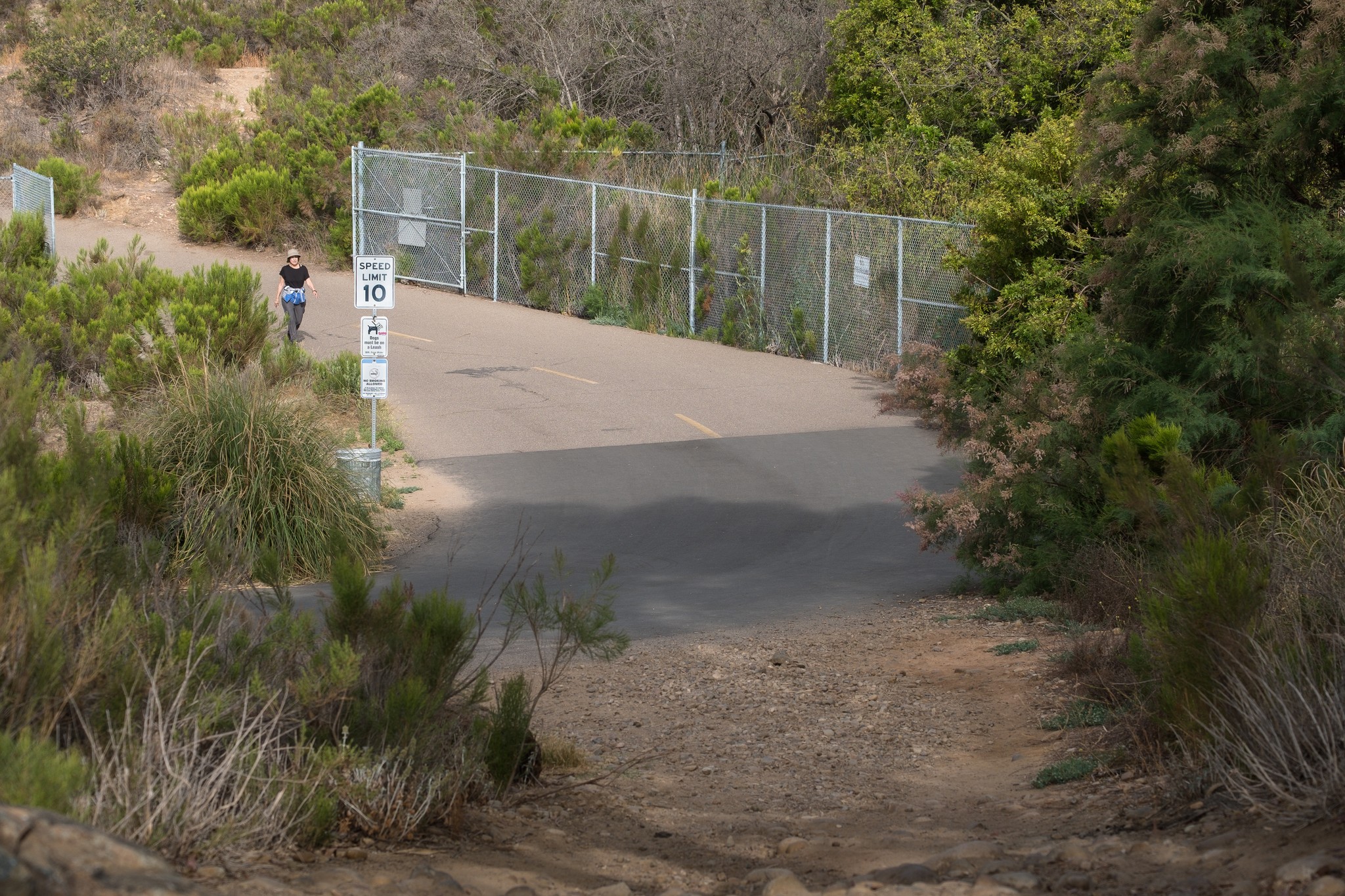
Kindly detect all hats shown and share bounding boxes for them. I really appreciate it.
[287,249,301,258]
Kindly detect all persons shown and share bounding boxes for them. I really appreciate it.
[274,249,319,346]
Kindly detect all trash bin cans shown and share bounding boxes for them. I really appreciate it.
[336,449,382,504]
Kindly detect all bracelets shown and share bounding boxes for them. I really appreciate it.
[312,290,317,293]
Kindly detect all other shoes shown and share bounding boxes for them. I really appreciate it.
[293,342,300,350]
